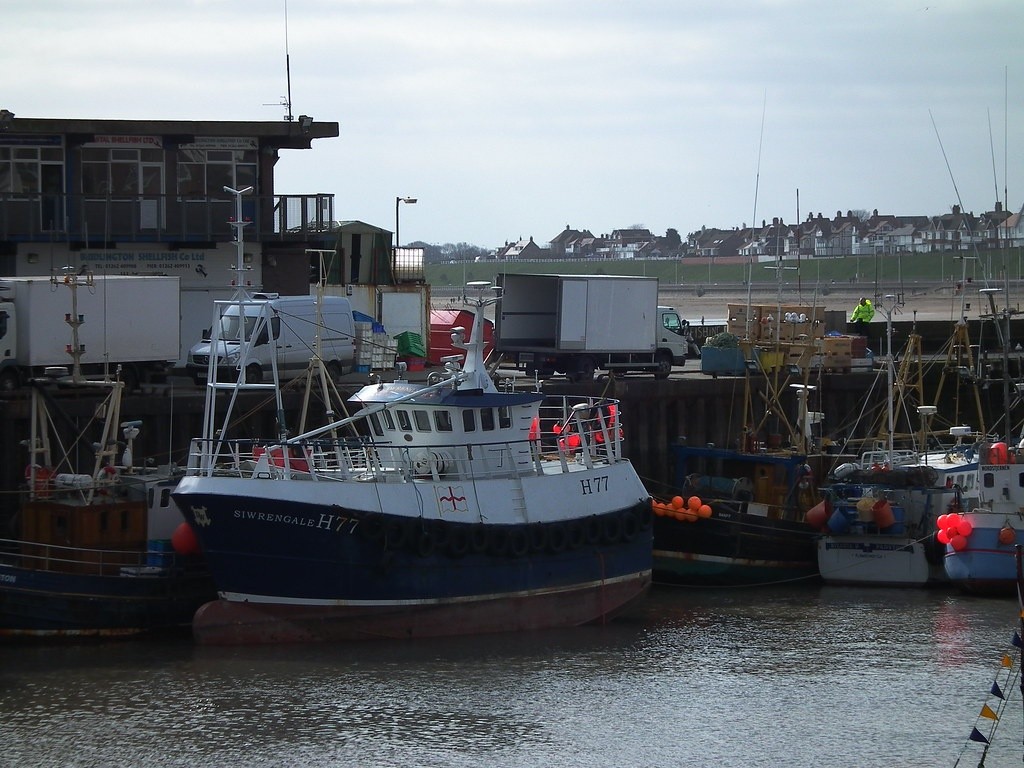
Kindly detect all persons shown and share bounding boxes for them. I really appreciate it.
[675,325,701,359]
[850,297,874,339]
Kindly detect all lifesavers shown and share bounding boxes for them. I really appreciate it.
[96,465,117,497]
[588,400,612,432]
[23,463,43,489]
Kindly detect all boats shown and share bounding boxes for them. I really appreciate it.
[943,309,1024,587]
[0,216,230,646]
[806,309,980,583]
[168,280,658,649]
[651,254,857,584]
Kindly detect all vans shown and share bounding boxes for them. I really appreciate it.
[185,296,358,390]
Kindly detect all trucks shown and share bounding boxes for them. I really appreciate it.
[494,272,691,383]
[0,276,182,390]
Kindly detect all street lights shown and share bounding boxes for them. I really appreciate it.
[396,197,417,247]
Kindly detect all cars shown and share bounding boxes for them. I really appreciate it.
[450,261,457,264]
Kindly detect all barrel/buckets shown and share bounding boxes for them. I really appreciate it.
[827,507,853,534]
[857,499,878,522]
[871,500,894,528]
[805,500,832,528]
